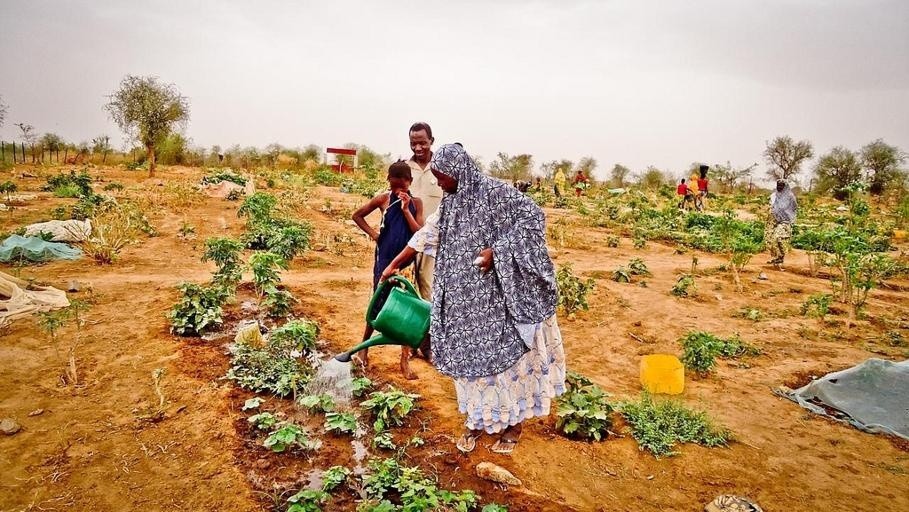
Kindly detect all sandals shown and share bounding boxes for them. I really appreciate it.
[399,349,433,365]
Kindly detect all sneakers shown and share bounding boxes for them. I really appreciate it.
[767,256,783,264]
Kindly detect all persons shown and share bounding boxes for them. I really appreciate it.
[379,143,567,453]
[676,172,708,212]
[516,179,532,192]
[571,170,586,197]
[352,158,425,380]
[536,177,541,191]
[764,179,799,264]
[554,168,566,189]
[398,122,443,364]
[545,175,548,184]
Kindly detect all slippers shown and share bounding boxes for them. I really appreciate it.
[491,433,522,453]
[456,432,483,453]
